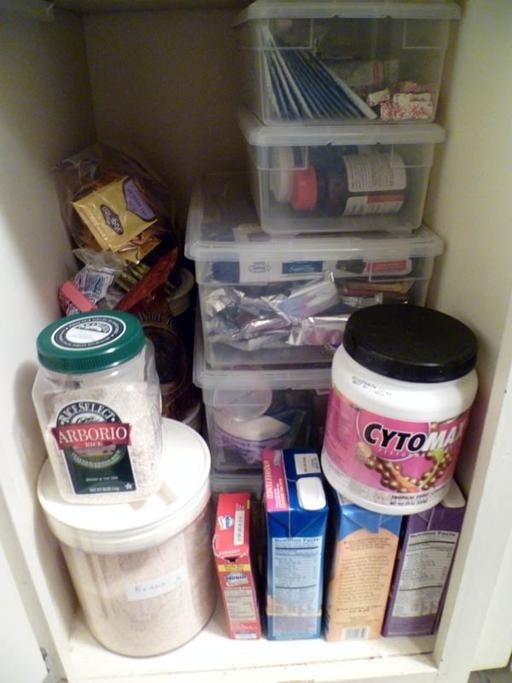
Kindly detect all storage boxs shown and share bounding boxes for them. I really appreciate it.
[179,0,465,514]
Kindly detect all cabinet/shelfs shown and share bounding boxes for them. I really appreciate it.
[1,1,512,683]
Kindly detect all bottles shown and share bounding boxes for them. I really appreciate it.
[272,141,405,218]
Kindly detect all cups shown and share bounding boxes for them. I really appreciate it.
[30,312,168,509]
